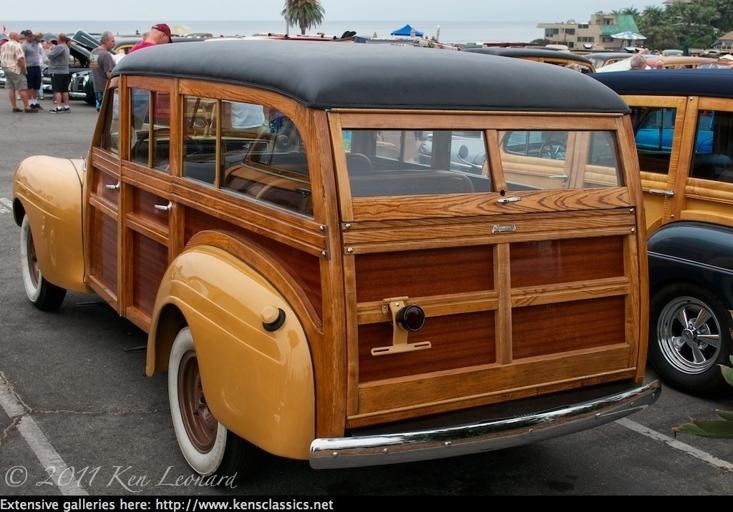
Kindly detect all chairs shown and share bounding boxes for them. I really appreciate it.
[343,151,474,195]
[695,153,731,179]
[166,157,218,183]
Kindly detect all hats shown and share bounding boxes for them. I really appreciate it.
[150,24,173,44]
[24,30,34,37]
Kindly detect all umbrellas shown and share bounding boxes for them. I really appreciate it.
[610,31,647,47]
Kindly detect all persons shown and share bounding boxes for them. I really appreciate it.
[630,54,652,69]
[128,23,172,122]
[231,99,265,131]
[0,30,71,114]
[90,30,115,111]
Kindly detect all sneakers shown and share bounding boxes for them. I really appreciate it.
[11,101,98,114]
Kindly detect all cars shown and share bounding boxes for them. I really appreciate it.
[0,30,733,485]
